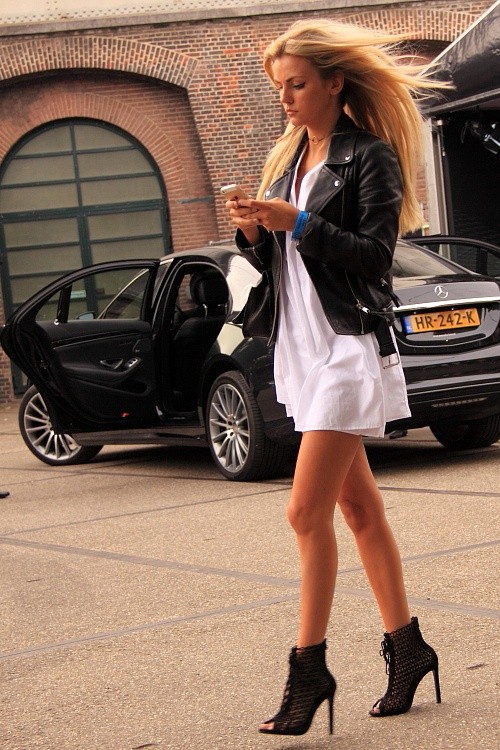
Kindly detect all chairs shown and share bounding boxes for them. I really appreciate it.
[171,271,229,392]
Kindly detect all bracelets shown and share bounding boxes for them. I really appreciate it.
[292,211,308,238]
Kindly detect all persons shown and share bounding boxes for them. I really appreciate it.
[225,16,442,736]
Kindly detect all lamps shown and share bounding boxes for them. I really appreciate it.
[461,120,500,155]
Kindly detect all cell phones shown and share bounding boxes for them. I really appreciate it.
[220,184,249,208]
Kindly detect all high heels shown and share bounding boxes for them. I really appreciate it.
[369,616,441,717]
[259,638,337,735]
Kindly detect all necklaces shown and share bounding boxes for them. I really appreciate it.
[308,135,327,144]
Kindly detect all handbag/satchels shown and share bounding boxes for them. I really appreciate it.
[232,270,272,339]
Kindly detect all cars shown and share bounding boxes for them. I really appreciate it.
[0,237,500,482]
